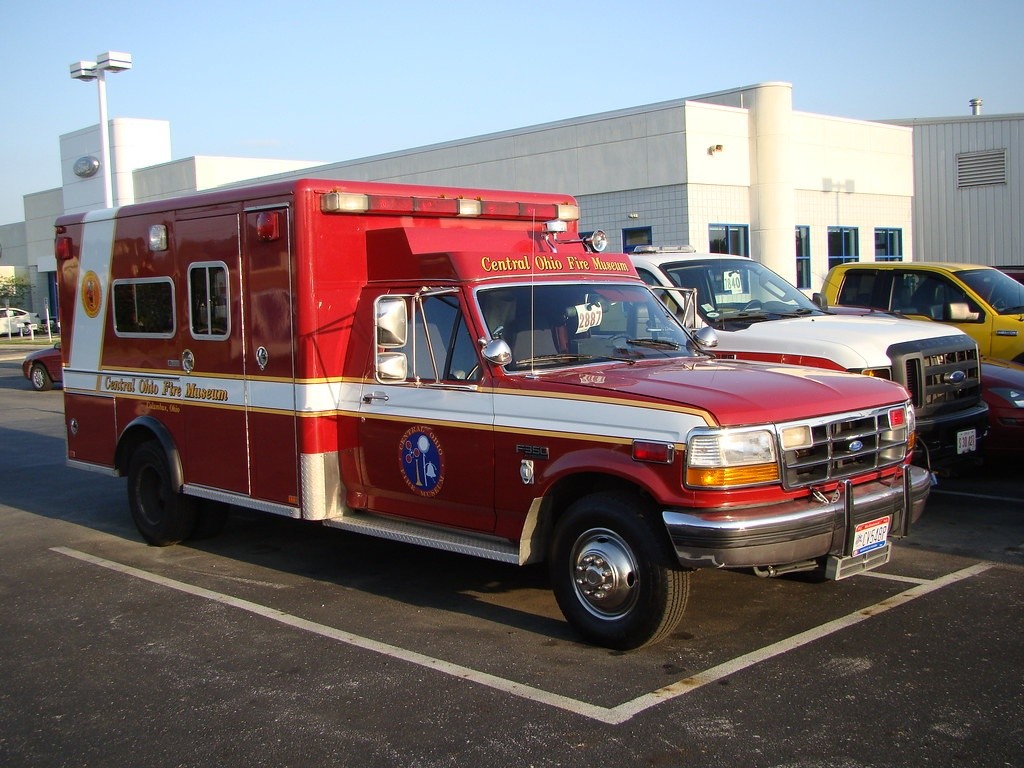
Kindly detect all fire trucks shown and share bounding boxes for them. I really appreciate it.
[56,178,938,655]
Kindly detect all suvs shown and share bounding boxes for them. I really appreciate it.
[578,241,994,499]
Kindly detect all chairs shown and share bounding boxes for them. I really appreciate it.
[927,285,947,321]
[895,285,919,315]
[512,290,558,367]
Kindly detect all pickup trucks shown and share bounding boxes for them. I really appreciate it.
[716,257,1024,364]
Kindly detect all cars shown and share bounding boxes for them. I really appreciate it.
[815,299,1023,440]
[23,342,62,391]
[0,307,42,336]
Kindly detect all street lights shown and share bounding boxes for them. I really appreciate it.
[69,51,133,208]
